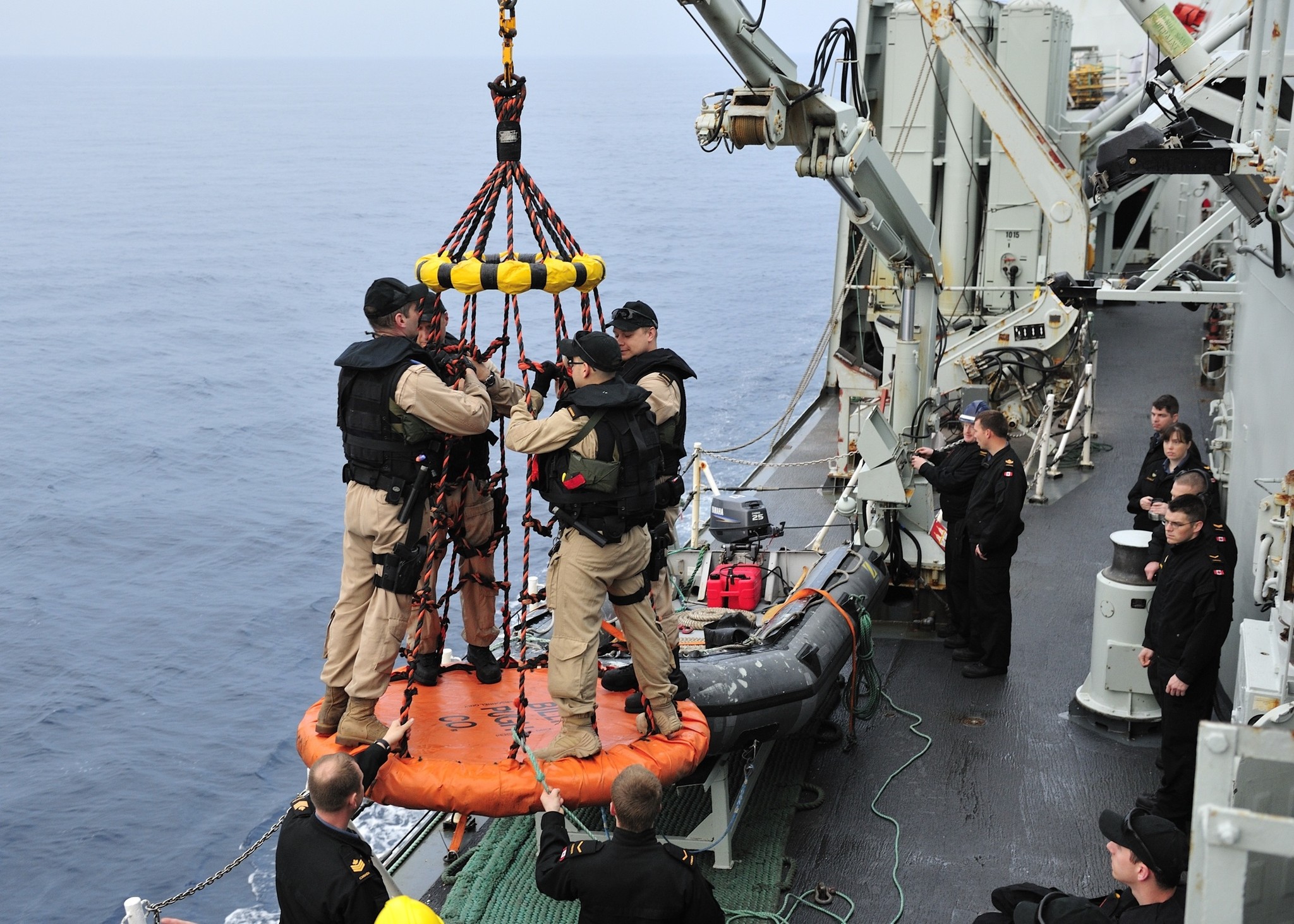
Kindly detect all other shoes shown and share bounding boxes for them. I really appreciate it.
[937,625,954,638]
[1135,793,1157,812]
[952,647,979,662]
[961,661,1007,679]
[943,632,969,649]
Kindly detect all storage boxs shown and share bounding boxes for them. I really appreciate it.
[709,494,770,544]
[706,561,761,609]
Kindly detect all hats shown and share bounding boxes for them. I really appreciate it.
[363,277,429,319]
[605,300,658,332]
[558,330,624,373]
[1097,806,1190,875]
[420,292,446,322]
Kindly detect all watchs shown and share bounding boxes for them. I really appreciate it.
[479,370,495,387]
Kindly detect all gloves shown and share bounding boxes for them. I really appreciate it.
[532,361,562,398]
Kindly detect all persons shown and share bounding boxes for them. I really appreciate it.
[505,300,691,761]
[275,716,414,924]
[535,764,726,924]
[912,400,1028,678]
[972,807,1186,924]
[314,277,526,747]
[1127,394,1238,833]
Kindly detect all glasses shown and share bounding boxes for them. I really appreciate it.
[611,308,658,328]
[567,359,596,372]
[572,330,597,364]
[1161,517,1199,529]
[1123,807,1163,875]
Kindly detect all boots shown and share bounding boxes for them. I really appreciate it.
[601,663,638,692]
[335,697,389,747]
[315,686,350,735]
[625,645,691,714]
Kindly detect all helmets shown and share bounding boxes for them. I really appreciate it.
[957,400,991,424]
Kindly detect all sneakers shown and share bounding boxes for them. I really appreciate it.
[466,644,502,684]
[414,653,441,686]
[635,701,683,736]
[532,712,602,762]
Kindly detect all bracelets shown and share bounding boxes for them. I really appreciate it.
[373,738,391,755]
